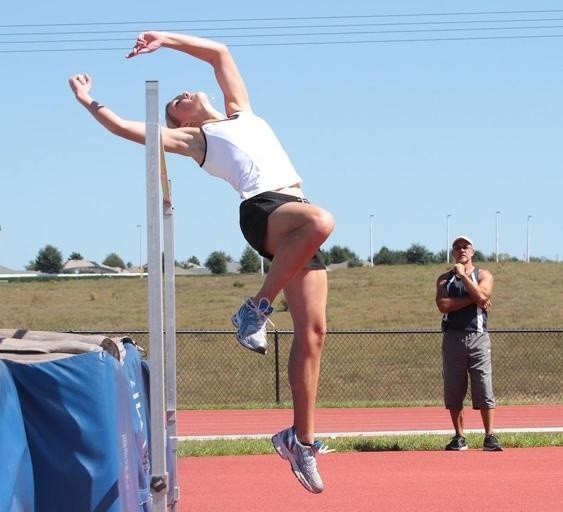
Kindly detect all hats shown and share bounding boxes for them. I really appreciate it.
[452,235,474,246]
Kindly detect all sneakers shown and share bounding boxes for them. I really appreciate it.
[480,434,505,452]
[445,434,470,452]
[270,423,325,495]
[229,295,278,356]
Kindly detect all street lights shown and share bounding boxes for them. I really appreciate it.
[135,223,143,271]
[369,213,376,267]
[526,214,533,262]
[444,213,452,264]
[495,210,502,263]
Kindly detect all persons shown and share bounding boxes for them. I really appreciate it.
[433,235,503,453]
[66,30,335,494]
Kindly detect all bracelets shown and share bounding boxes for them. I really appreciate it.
[84,98,104,117]
